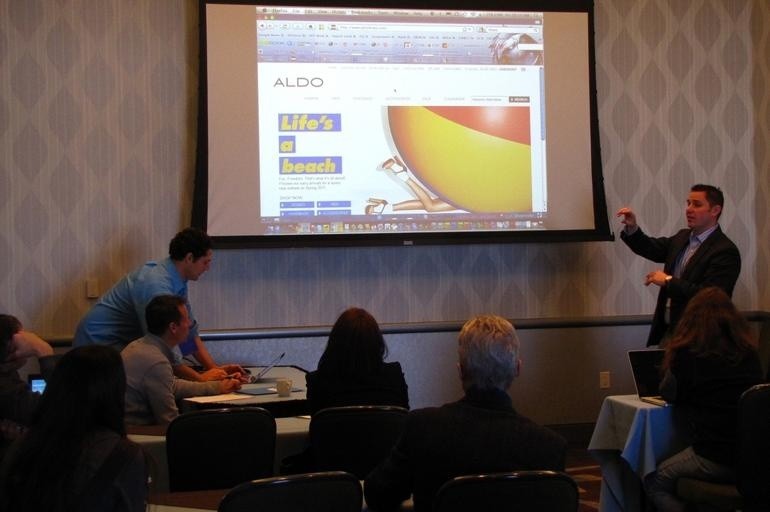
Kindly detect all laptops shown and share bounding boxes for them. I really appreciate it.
[627,349,672,408]
[232,352,286,383]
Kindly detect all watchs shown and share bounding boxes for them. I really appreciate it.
[664,275,673,284]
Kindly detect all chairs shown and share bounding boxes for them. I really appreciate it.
[687,381,770,498]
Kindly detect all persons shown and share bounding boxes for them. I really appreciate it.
[640,284,766,511]
[119,293,241,426]
[0,313,64,469]
[1,344,151,511]
[282,306,412,477]
[70,225,246,381]
[363,313,573,512]
[616,182,742,347]
[364,155,460,216]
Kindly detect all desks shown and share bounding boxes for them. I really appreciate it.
[586,393,686,512]
[140,476,412,512]
[181,378,308,408]
[121,415,312,488]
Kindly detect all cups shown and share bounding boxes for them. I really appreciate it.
[276,378,293,397]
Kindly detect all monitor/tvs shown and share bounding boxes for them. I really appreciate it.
[28,374,47,395]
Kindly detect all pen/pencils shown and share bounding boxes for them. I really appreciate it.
[223,375,235,378]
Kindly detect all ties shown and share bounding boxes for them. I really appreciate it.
[663,243,700,326]
